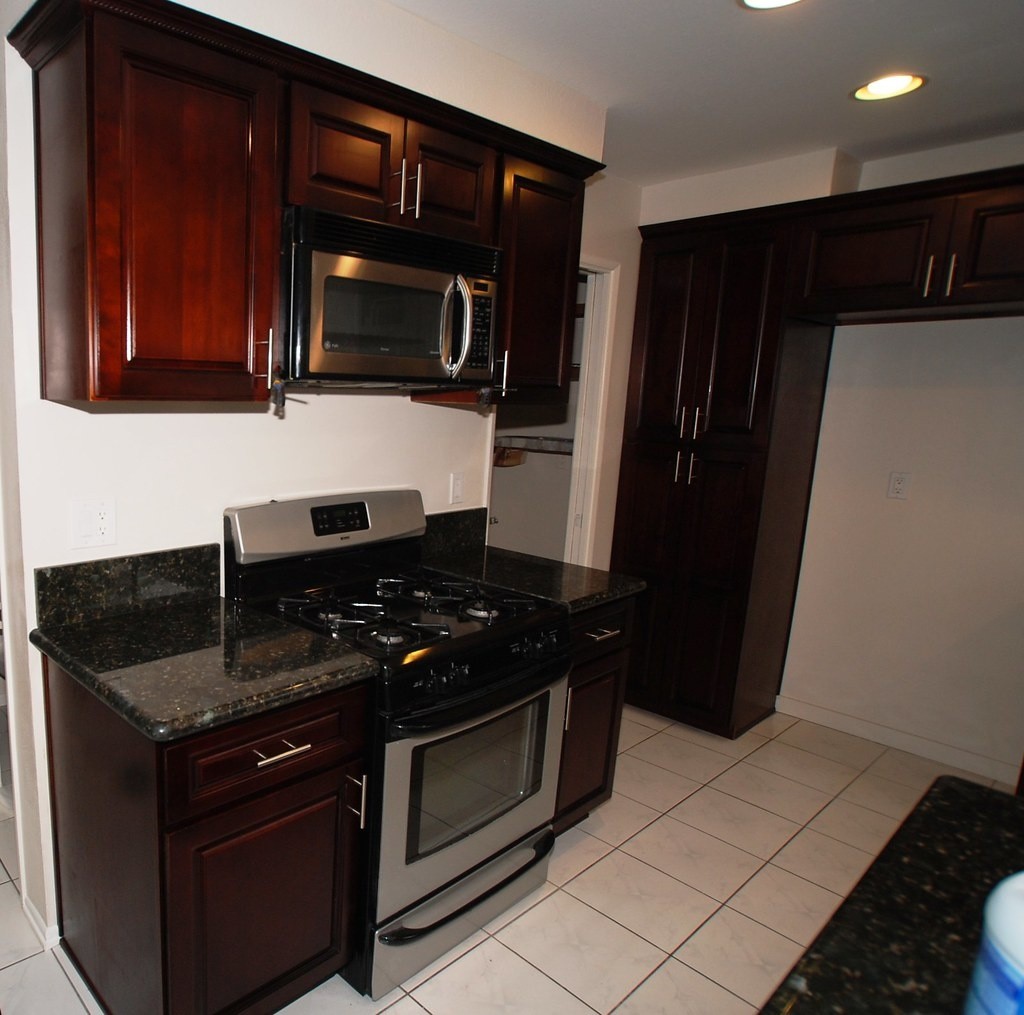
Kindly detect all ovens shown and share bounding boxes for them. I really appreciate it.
[338,625,573,1003]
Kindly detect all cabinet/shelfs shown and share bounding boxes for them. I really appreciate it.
[7,0,607,403]
[42,656,355,1014]
[548,593,637,840]
[608,163,1024,740]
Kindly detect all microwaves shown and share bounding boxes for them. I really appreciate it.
[280,209,508,400]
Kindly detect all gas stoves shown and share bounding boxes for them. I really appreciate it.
[263,565,567,670]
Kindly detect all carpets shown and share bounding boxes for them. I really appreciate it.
[753,772,1024,1015]
